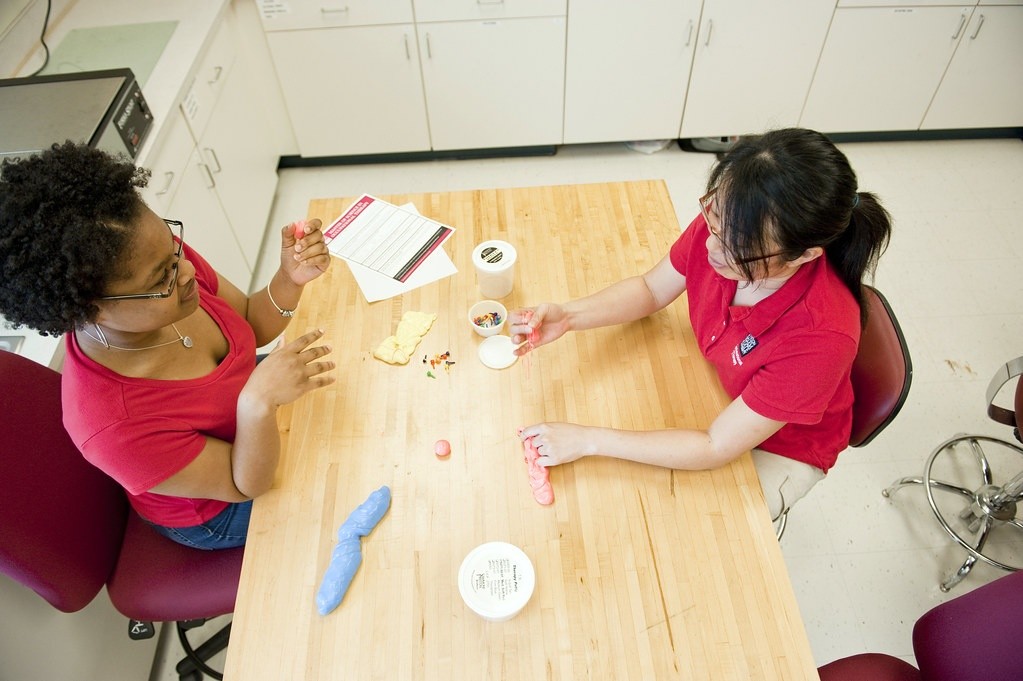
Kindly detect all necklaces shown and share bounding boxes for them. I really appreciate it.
[79,321,194,350]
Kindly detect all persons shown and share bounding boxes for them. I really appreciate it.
[0,137,337,548]
[508,127,892,521]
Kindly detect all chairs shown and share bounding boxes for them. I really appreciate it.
[774,286,914,544]
[816,569,1023,681]
[0,349,246,681]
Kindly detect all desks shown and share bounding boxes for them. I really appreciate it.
[221,176,822,681]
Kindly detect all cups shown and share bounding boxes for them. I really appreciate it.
[471,240,518,299]
[458,542,534,622]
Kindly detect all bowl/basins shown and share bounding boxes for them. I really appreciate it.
[469,300,508,338]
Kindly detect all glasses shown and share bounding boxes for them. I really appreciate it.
[95,218,184,301]
[698,185,799,265]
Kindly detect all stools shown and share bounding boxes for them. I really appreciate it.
[880,357,1023,593]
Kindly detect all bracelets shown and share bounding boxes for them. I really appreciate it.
[267,283,300,317]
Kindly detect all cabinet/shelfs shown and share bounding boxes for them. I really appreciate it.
[143,0,1023,296]
